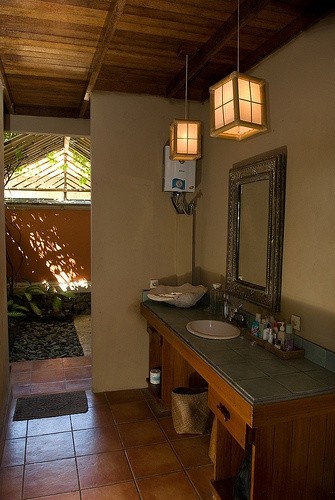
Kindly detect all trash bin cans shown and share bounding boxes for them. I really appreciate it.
[171,386,209,436]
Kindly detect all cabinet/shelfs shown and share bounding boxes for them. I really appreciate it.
[139,303,335,500]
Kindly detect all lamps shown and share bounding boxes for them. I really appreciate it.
[170,56,202,162]
[209,0,272,142]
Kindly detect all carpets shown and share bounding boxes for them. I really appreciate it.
[13,390,88,421]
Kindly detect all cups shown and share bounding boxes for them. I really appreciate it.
[150,370,161,384]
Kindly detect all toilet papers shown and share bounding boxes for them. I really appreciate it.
[149,368,161,385]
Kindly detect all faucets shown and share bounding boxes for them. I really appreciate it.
[232,308,250,325]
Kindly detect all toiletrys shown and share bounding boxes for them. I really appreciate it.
[248,312,305,360]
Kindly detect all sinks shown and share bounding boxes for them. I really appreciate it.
[185,318,241,340]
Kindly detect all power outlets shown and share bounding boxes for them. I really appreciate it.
[291,314,300,330]
[150,280,159,289]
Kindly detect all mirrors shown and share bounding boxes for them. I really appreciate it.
[225,153,286,313]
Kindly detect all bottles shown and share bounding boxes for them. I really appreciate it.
[286,324,293,350]
[209,283,223,313]
